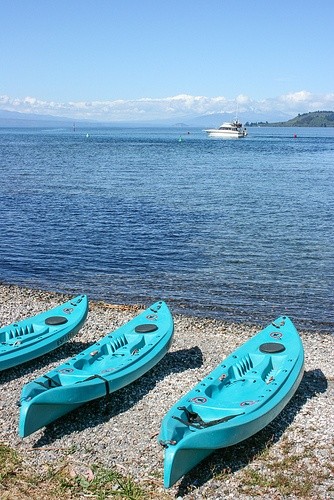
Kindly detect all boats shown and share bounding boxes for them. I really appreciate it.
[156,314,307,489]
[15,300,175,439]
[0,293,89,373]
[202,121,249,138]
